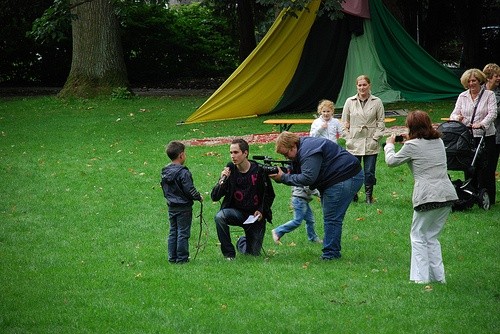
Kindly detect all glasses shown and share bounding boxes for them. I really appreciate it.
[284,150,289,157]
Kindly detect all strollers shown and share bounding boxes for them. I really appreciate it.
[435,120,491,211]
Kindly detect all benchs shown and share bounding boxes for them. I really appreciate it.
[263,118,396,133]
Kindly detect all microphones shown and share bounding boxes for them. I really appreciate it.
[253,155,273,160]
[221,162,234,181]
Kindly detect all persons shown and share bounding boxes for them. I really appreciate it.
[449,68,497,204]
[383,110,459,284]
[340,75,386,204]
[271,181,324,246]
[211,139,276,260]
[269,130,365,259]
[309,100,350,146]
[481,63,500,160]
[160,141,204,264]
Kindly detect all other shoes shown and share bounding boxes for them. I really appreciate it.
[415,280,429,284]
[316,239,323,243]
[272,229,280,244]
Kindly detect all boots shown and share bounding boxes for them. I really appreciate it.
[366,190,371,204]
[354,194,358,201]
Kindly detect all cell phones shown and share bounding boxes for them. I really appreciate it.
[395,136,404,142]
